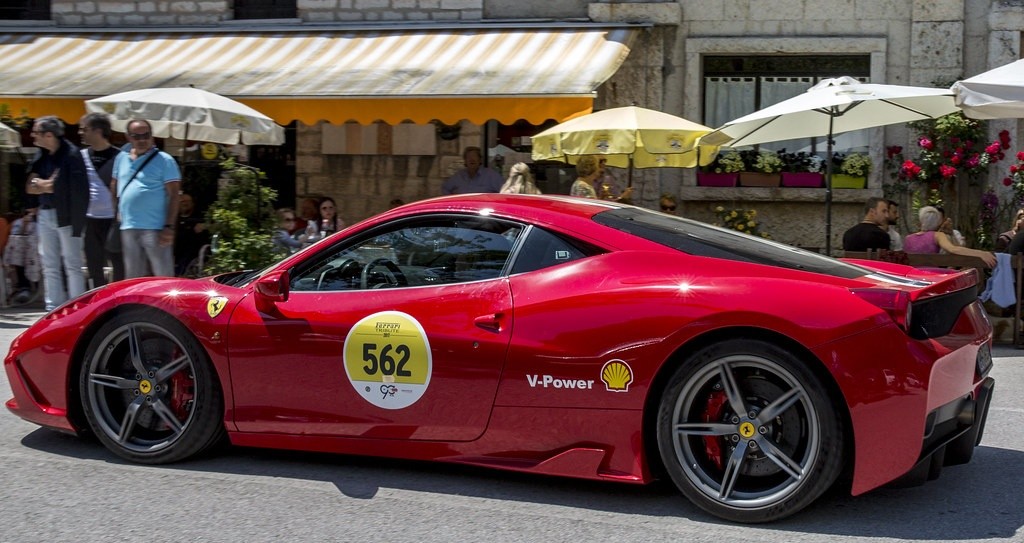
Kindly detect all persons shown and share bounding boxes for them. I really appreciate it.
[441,147,1024,270]
[453,232,512,280]
[0,113,405,323]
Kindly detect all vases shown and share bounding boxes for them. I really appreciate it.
[697,170,739,187]
[780,172,824,187]
[739,172,781,186]
[831,174,866,188]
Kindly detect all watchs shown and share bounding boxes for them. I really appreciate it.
[31,178,38,189]
[617,196,623,202]
[164,224,176,230]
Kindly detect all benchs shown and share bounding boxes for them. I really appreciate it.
[834,248,1022,350]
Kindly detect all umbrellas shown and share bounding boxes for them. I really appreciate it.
[82,84,284,192]
[530,62,1023,260]
[0,122,22,149]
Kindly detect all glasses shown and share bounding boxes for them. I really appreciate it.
[32,130,51,136]
[279,218,295,222]
[80,127,98,133]
[131,132,150,140]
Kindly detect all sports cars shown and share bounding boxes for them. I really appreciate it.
[2,193,995,522]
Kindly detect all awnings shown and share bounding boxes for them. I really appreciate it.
[0,27,644,126]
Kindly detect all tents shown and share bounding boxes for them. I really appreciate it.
[488,144,534,167]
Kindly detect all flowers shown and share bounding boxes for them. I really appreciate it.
[752,152,786,173]
[832,153,874,176]
[700,148,747,174]
[712,206,773,240]
[885,130,1024,210]
[780,152,826,174]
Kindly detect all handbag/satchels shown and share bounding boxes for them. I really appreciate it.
[104,226,122,253]
